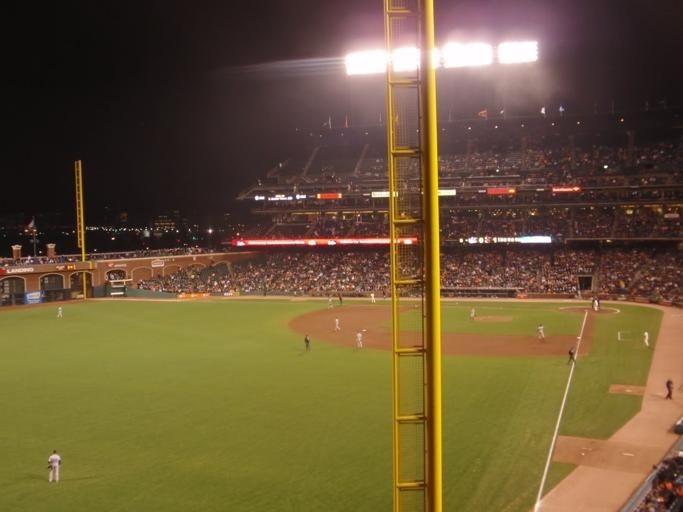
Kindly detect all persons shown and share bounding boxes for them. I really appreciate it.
[634,448,682,511]
[567,347,576,364]
[1,245,205,271]
[536,323,545,343]
[47,449,63,483]
[354,330,362,350]
[643,329,649,346]
[334,317,340,332]
[56,305,62,321]
[664,379,674,400]
[126,105,683,312]
[304,333,311,353]
[469,307,475,321]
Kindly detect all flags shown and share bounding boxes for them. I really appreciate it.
[28,219,33,229]
